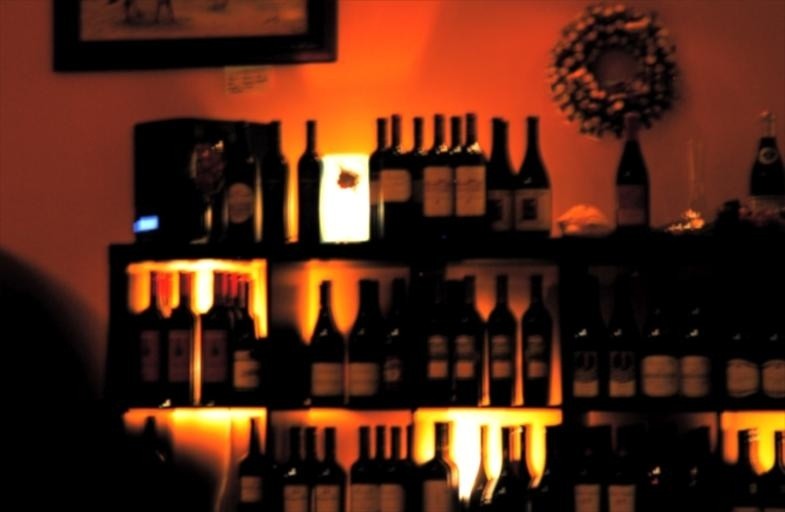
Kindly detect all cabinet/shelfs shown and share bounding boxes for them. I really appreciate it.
[107,242,785,433]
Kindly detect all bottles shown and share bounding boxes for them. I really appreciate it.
[312,427,345,511]
[423,420,460,511]
[371,423,388,476]
[568,283,785,403]
[748,109,785,217]
[259,118,291,242]
[226,120,257,244]
[401,421,420,511]
[570,424,601,512]
[538,426,570,511]
[383,424,404,509]
[236,415,275,511]
[302,424,319,479]
[297,118,324,241]
[277,424,308,508]
[369,113,553,240]
[135,269,259,396]
[604,423,639,510]
[185,143,213,244]
[349,420,376,509]
[491,424,520,510]
[614,112,651,228]
[305,269,552,407]
[141,412,158,445]
[466,423,492,509]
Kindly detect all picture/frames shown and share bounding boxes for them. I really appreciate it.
[51,0,338,74]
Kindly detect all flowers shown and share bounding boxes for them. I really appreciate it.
[547,6,682,137]
